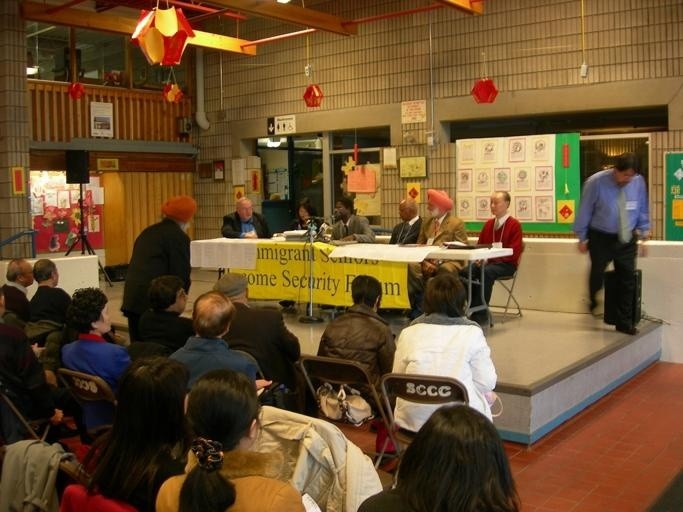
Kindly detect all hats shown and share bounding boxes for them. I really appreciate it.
[213,272,248,296]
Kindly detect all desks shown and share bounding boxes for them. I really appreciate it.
[188,237,513,327]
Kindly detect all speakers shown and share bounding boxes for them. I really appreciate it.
[604,269,643,325]
[55,47,81,82]
[66,150,90,185]
[178,117,192,134]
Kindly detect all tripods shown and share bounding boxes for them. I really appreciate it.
[64,183,114,287]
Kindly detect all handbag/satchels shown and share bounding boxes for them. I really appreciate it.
[315,382,375,427]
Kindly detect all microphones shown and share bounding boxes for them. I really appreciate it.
[330,213,342,219]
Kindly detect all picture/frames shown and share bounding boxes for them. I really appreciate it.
[198,163,212,178]
[213,160,225,181]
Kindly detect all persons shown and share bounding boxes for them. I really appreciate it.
[355,404,521,512]
[167,290,265,392]
[388,197,422,244]
[0,257,34,320]
[317,275,397,415]
[211,272,301,413]
[220,196,272,239]
[573,152,651,335]
[75,355,189,511]
[277,197,319,308]
[152,367,307,512]
[469,190,522,324]
[390,274,498,439]
[0,291,73,424]
[61,287,134,430]
[135,275,195,350]
[120,195,197,344]
[407,188,468,320]
[328,196,375,244]
[23,259,119,344]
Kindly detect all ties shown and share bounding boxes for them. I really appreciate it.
[344,225,348,236]
[616,188,631,243]
[434,220,439,232]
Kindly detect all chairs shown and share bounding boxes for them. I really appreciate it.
[299,355,400,471]
[55,369,117,443]
[230,349,280,402]
[380,372,470,491]
[495,242,527,323]
[0,444,93,512]
[1,381,57,442]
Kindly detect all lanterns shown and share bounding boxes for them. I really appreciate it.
[131,7,195,67]
